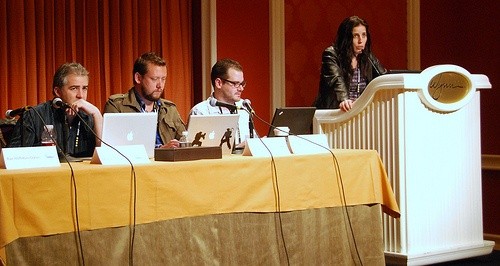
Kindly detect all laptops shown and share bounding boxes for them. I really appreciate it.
[268,107,316,138]
[101,112,158,161]
[185,115,238,156]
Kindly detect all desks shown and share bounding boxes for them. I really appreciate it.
[0,149,401,266]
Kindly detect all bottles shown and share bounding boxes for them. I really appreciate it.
[179,131,192,148]
[41,125,57,146]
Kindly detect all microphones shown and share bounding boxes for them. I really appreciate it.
[54,98,71,109]
[210,98,236,110]
[361,49,381,76]
[5,105,30,119]
[242,99,255,114]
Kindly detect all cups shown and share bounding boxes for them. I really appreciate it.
[274,126,290,137]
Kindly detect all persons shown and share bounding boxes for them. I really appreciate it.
[6,62,103,161]
[185,58,258,143]
[308,16,384,134]
[102,52,189,148]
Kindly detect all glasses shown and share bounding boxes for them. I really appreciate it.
[224,79,246,88]
